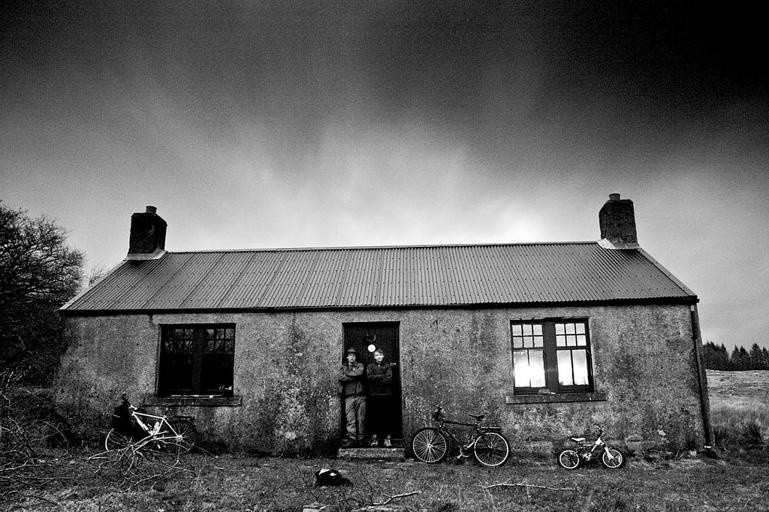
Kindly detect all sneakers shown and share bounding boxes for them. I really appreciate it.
[342,434,392,448]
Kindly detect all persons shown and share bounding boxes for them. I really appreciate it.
[337,346,367,448]
[365,348,393,447]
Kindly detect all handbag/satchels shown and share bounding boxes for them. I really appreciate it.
[314,470,353,489]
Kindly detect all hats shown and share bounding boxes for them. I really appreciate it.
[347,348,356,352]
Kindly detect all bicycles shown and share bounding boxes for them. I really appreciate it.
[104,393,198,455]
[556,423,625,470]
[411,404,511,469]
